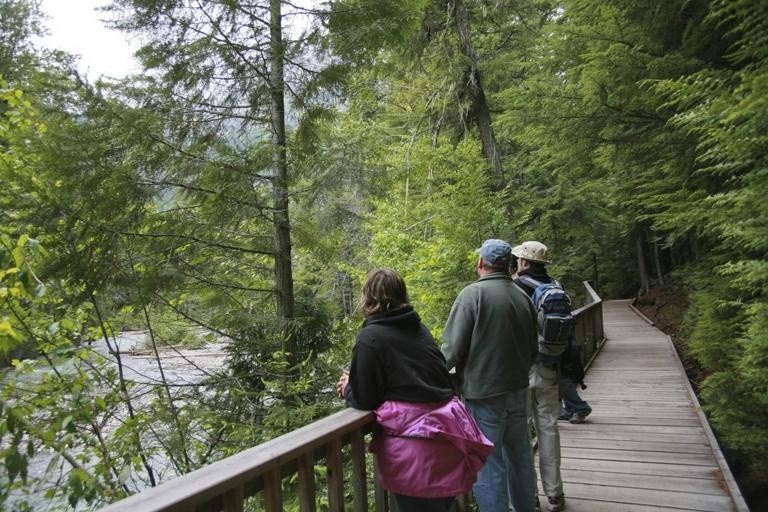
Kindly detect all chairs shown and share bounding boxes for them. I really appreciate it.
[558,400,592,424]
[532,495,565,512]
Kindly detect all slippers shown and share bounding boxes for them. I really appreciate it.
[518,275,573,367]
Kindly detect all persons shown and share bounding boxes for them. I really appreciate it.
[509,241,565,512]
[336,266,495,512]
[441,239,540,511]
[509,255,592,424]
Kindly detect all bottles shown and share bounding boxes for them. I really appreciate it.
[511,240,551,264]
[475,238,513,265]
